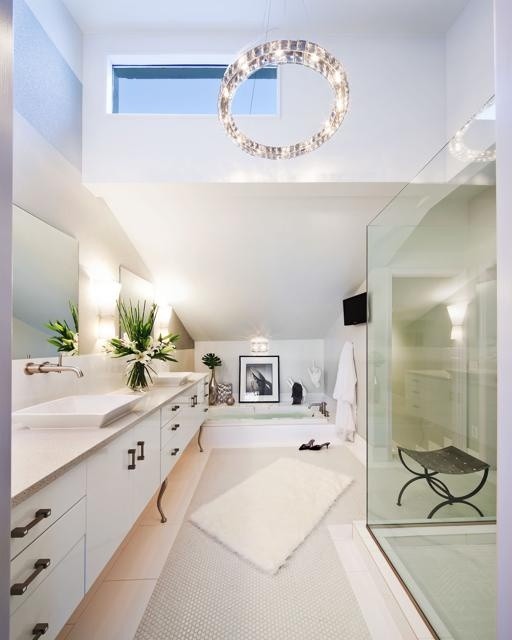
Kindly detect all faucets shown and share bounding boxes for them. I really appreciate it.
[24,362,84,378]
[308,402,329,417]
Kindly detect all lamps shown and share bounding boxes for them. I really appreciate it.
[217,2,354,162]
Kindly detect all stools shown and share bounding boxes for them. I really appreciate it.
[395,444,491,520]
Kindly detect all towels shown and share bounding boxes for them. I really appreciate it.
[333,341,358,442]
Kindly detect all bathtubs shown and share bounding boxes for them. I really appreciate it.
[208,403,330,424]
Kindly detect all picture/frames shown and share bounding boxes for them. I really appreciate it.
[239,355,280,403]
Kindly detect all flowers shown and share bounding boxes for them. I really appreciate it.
[40,296,78,359]
[97,296,182,388]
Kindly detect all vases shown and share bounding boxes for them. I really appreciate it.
[122,359,155,392]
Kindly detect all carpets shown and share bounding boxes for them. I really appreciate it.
[133,441,373,638]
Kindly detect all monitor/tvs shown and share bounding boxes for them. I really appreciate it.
[343,292,366,325]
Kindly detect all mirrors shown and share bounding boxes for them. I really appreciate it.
[11,203,80,362]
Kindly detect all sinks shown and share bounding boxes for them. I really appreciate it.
[12,393,142,428]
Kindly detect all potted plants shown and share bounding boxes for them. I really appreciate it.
[202,353,222,405]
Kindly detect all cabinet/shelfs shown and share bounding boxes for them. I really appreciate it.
[186,377,209,442]
[161,393,187,483]
[85,409,161,593]
[10,463,84,640]
